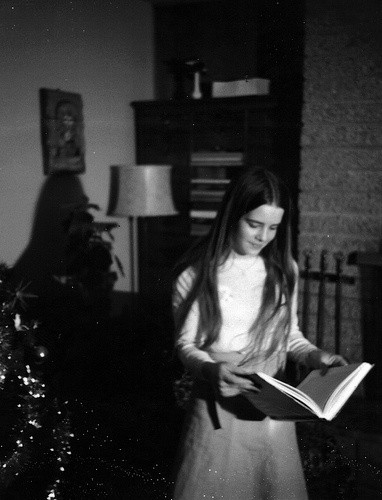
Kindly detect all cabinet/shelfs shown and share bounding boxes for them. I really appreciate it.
[129,96,281,293]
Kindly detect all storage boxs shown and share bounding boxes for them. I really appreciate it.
[212,79,270,97]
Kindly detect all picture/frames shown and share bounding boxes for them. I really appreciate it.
[39,87,85,175]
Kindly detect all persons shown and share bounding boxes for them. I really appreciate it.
[170,167,349,500]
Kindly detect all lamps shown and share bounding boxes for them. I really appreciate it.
[106,165,180,293]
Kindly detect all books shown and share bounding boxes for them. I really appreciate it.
[238,362,376,422]
